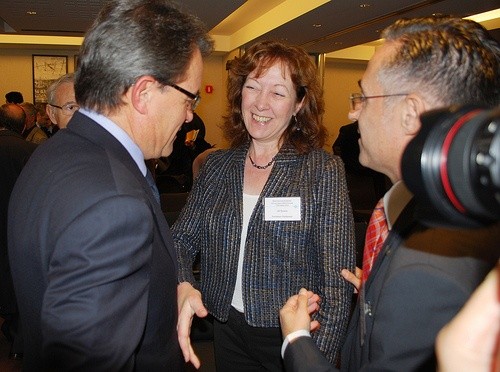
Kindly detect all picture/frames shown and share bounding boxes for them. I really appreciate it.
[32,54,69,105]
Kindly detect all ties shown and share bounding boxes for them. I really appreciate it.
[361,197,387,287]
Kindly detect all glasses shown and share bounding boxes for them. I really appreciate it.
[49,103,77,116]
[349,93,407,111]
[170,84,200,111]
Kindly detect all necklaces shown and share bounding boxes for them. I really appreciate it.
[248,154,277,169]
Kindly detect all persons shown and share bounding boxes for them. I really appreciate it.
[153,112,211,192]
[331,79,393,270]
[280,16,500,372]
[435,261,500,372]
[0,73,81,218]
[177,37,357,372]
[0,0,214,372]
